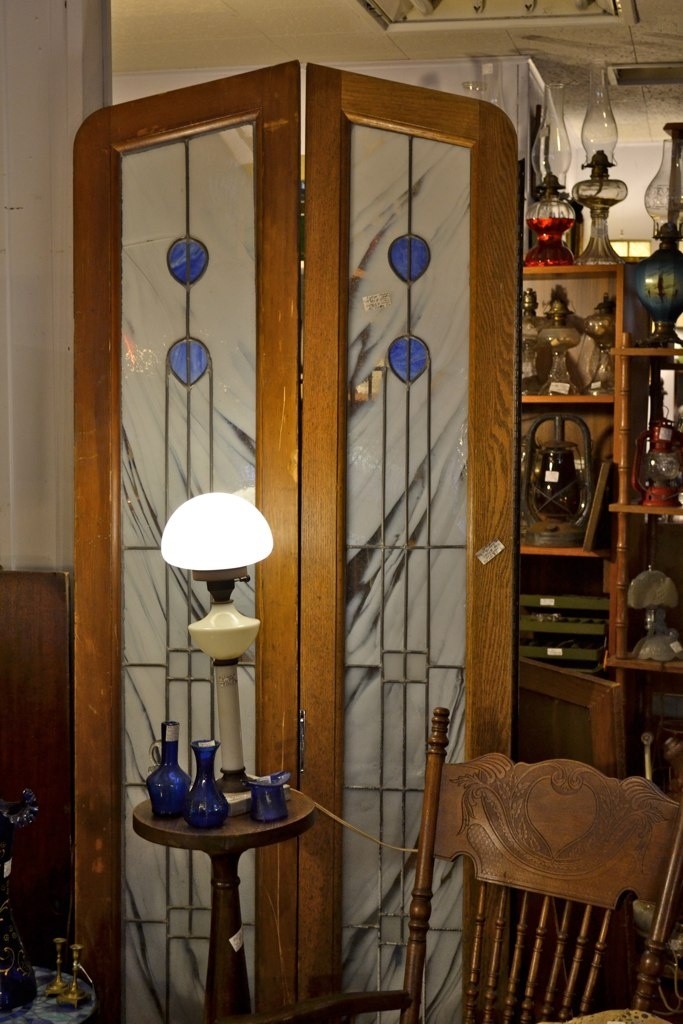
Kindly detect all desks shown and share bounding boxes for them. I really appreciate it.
[0,966,96,1024]
[132,784,313,1024]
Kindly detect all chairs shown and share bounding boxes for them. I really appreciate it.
[216,707,683,1024]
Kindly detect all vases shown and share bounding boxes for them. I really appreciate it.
[0,787,38,1011]
[146,720,192,817]
[183,739,228,828]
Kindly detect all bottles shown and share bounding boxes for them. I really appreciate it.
[0,788,39,1011]
[145,721,192,821]
[183,739,229,830]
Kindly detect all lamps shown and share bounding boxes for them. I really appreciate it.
[160,492,275,793]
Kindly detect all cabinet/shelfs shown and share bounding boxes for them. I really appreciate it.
[74,49,528,1024]
[514,262,683,982]
